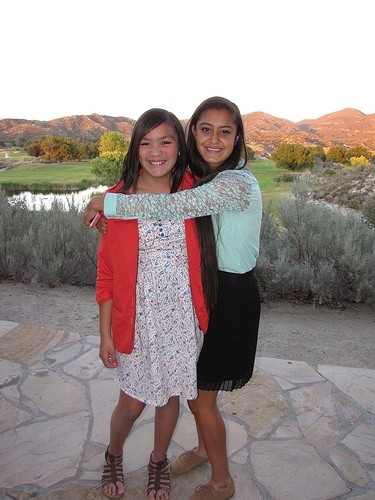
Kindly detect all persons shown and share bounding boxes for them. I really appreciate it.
[80,93,262,500]
[93,107,213,500]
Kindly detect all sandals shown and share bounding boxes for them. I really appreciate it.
[101,444,125,498]
[147,450,171,500]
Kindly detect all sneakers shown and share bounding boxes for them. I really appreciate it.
[171,446,210,475]
[187,477,235,500]
